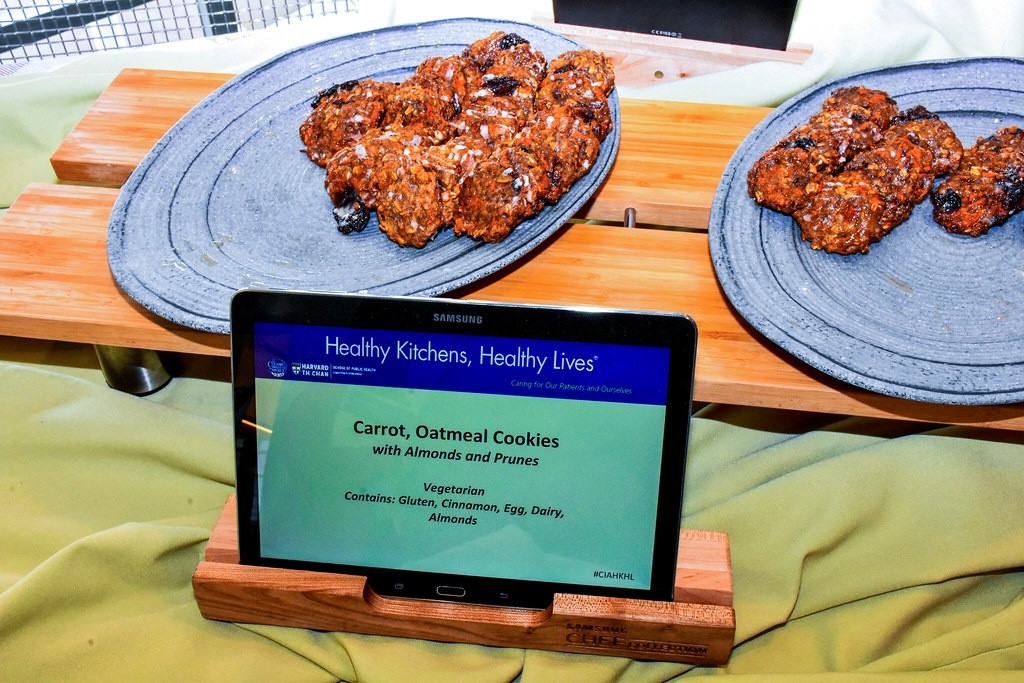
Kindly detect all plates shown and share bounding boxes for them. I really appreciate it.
[106,18,620,335]
[707,57,1024,406]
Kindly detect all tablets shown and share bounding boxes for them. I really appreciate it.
[229,286,700,610]
